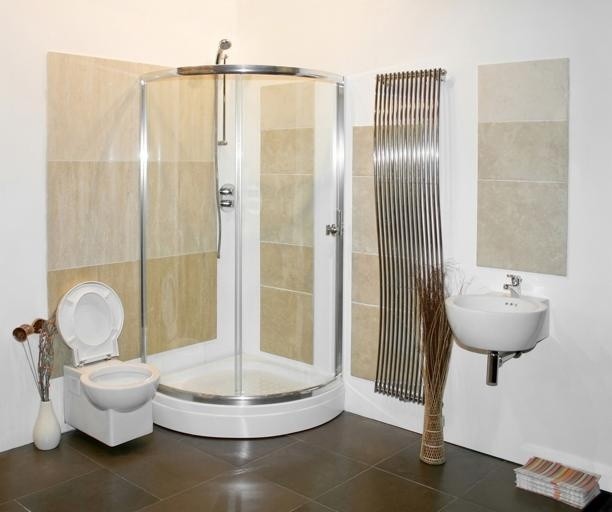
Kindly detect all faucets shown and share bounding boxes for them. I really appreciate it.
[503,273,522,290]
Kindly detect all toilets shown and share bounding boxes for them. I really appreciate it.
[56,279,162,446]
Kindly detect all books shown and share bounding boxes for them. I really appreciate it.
[511,454,602,509]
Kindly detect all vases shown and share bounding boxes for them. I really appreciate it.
[418,387,450,468]
[31,395,61,450]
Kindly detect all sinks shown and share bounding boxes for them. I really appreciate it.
[442,293,546,351]
